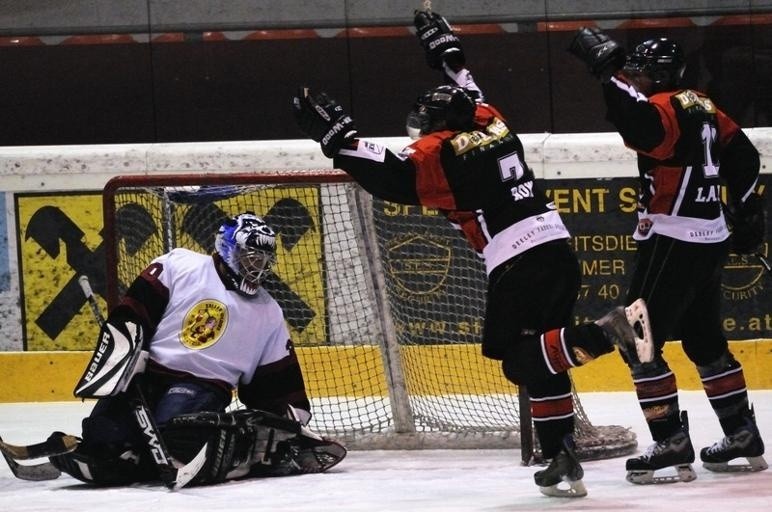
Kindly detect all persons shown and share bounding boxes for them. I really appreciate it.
[568,19,768,471]
[288,8,639,486]
[45,210,312,489]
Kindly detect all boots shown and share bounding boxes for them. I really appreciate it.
[626,410,695,471]
[595,305,641,365]
[700,402,765,463]
[534,453,583,487]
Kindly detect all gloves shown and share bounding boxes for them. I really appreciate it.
[413,8,465,74]
[293,86,358,159]
[568,25,625,76]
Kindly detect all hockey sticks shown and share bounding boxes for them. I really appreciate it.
[77,274,208,492]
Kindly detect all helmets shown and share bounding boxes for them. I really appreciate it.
[621,36,686,98]
[413,84,478,140]
[215,212,277,277]
